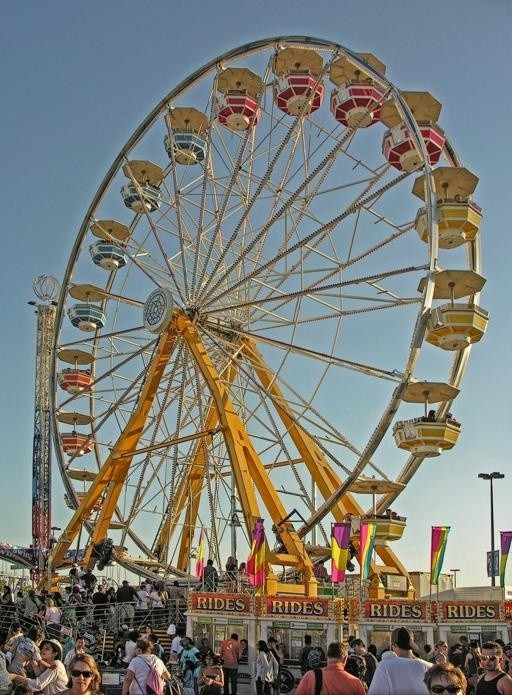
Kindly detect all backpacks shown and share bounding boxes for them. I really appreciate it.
[429,651,441,664]
[134,654,164,695]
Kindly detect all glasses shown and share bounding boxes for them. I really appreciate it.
[480,655,501,661]
[429,684,462,695]
[71,669,94,679]
[440,645,447,648]
[458,647,463,651]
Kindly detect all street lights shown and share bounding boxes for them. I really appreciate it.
[51,525,62,538]
[476,471,504,590]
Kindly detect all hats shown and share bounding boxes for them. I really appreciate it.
[195,649,224,666]
[41,638,63,660]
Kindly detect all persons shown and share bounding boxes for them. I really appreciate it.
[429,410,435,418]
[456,194,462,200]
[72,431,77,437]
[386,509,397,515]
[446,413,452,419]
